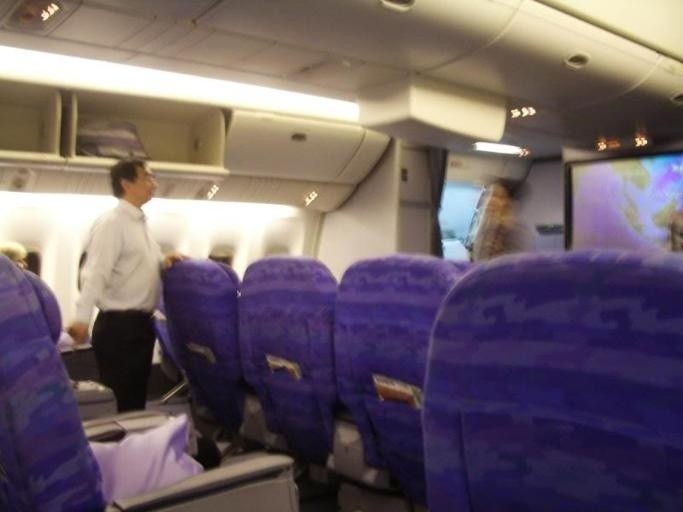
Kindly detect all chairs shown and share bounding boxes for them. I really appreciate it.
[21,269,117,443]
[151,280,189,404]
[333,254,463,508]
[163,258,241,459]
[2,251,301,511]
[237,257,338,484]
[421,250,682,512]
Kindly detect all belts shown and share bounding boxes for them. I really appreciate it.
[95,308,153,318]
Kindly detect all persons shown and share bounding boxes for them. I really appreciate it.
[469,174,539,265]
[87,411,339,511]
[65,156,191,414]
[663,211,682,253]
[0,240,31,270]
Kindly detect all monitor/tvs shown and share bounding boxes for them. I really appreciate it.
[563,150,683,254]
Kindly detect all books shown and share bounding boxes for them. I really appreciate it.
[371,371,423,411]
[184,341,214,366]
[264,353,301,379]
[150,308,167,326]
